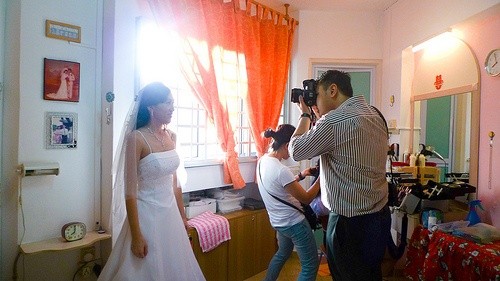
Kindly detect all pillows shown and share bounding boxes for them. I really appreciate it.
[454,222,500,243]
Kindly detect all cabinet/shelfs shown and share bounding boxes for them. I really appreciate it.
[189,206,276,281]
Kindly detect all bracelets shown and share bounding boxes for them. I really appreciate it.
[301,113,313,120]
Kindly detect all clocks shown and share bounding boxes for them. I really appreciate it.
[484,48,500,76]
[61,221,87,242]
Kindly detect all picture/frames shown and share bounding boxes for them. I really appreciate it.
[43,57,80,102]
[308,57,382,110]
[44,112,79,149]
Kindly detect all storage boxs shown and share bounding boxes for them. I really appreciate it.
[184,201,216,218]
[214,195,245,213]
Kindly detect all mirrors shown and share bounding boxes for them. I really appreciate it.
[409,83,480,213]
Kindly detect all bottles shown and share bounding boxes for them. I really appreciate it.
[428,210,437,232]
[403,150,425,167]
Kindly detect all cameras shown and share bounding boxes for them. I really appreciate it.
[291,79,318,107]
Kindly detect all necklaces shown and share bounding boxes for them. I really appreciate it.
[149,128,166,147]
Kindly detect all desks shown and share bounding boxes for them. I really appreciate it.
[396,224,500,281]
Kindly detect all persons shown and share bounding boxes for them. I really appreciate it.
[97,82,206,281]
[46,67,75,100]
[55,125,69,144]
[256,70,391,281]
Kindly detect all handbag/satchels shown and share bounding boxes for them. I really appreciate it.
[302,203,318,232]
[388,181,399,206]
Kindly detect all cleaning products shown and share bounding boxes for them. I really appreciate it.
[464,199,485,227]
[427,210,437,231]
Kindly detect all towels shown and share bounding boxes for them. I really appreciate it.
[186,209,232,254]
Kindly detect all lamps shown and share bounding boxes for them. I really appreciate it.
[412,27,452,52]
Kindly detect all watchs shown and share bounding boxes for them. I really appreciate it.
[298,172,305,180]
